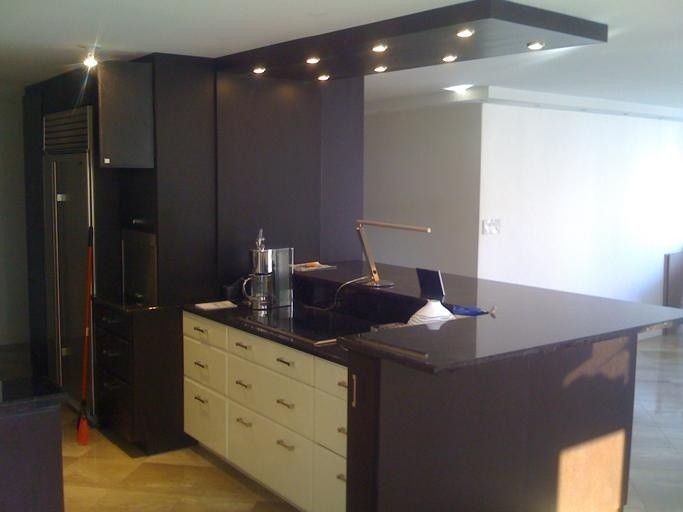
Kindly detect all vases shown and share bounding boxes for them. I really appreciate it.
[404,297,458,329]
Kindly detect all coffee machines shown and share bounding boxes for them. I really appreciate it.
[240,247,295,310]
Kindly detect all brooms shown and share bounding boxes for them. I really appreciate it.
[74,224,95,447]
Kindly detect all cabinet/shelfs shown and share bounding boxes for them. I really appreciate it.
[92,51,215,173]
[20,90,51,376]
[313,356,350,512]
[182,310,228,463]
[227,325,313,510]
[92,294,198,456]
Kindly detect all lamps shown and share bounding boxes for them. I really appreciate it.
[353,216,433,289]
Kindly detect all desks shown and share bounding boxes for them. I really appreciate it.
[1,341,71,512]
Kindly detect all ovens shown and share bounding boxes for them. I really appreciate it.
[117,229,157,308]
